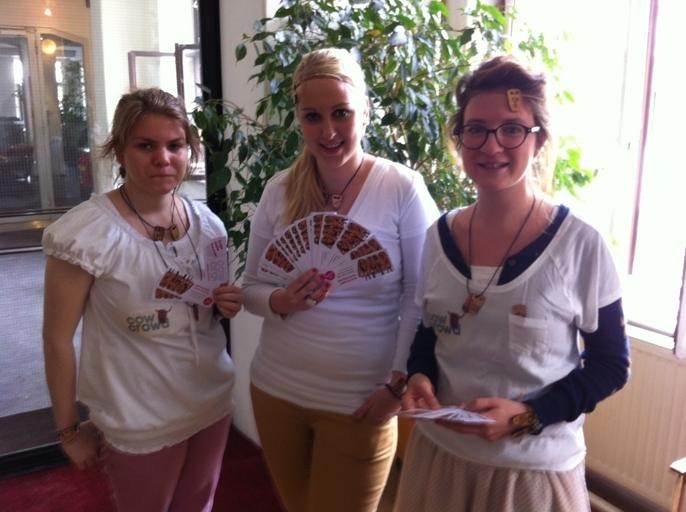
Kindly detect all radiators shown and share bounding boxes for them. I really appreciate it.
[580,323,686,511]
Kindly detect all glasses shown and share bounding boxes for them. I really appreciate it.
[453,122,542,150]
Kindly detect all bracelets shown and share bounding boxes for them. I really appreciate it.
[512,400,543,439]
[53,421,83,441]
[384,382,401,400]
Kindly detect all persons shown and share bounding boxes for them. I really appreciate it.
[40,85,247,510]
[389,56,633,512]
[239,45,441,512]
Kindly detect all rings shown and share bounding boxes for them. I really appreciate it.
[304,296,318,308]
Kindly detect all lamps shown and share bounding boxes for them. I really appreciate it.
[42,38,57,54]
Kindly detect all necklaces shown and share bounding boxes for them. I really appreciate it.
[118,186,181,242]
[461,192,537,317]
[313,156,366,211]
[122,187,205,322]
